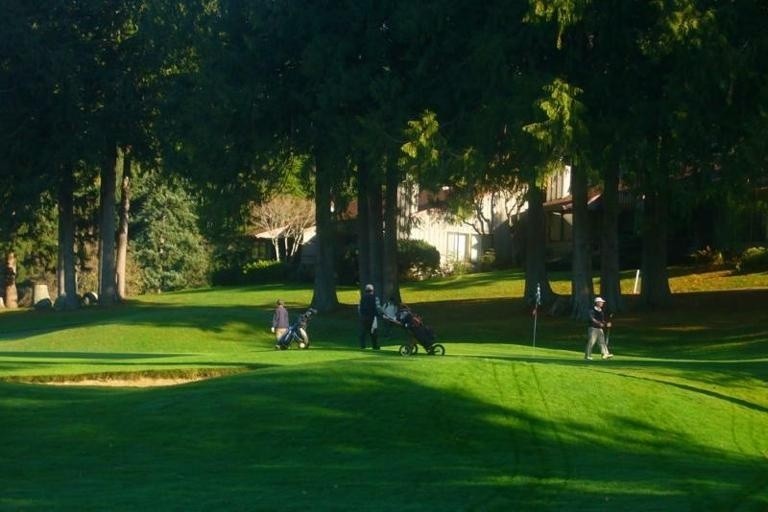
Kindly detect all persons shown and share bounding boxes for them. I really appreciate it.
[358,283,385,350]
[584,296,613,360]
[271,299,289,341]
[383,295,397,338]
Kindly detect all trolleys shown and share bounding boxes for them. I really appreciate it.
[275,308,318,350]
[382,303,445,356]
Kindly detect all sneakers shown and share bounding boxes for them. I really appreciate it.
[585,354,613,360]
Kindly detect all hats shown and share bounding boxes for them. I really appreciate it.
[365,284,374,291]
[594,297,606,303]
